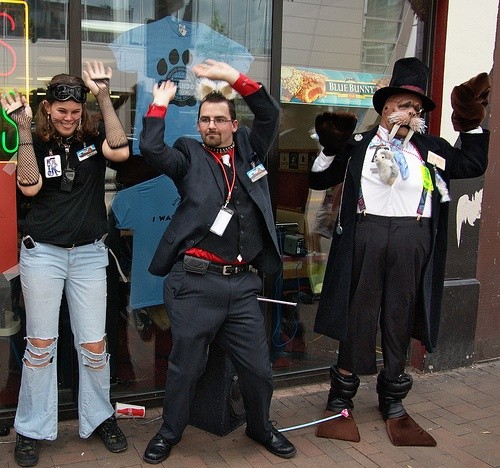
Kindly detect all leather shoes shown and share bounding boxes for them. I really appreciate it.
[142,433,171,464]
[245,420,297,458]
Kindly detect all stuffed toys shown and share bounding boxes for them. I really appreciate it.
[374,149,399,185]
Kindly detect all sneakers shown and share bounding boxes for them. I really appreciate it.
[95,412,129,453]
[14,433,39,466]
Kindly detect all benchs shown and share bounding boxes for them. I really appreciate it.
[275,207,305,279]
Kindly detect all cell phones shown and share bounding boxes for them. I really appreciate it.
[60,169,75,193]
[23,235,36,249]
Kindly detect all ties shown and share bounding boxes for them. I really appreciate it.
[222,153,231,167]
[390,139,409,179]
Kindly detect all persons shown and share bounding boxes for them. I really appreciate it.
[138,58,297,463]
[1,59,130,467]
[309,181,345,239]
[308,57,491,447]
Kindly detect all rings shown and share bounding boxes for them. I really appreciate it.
[15,97,20,101]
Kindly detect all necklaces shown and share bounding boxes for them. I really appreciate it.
[202,142,235,153]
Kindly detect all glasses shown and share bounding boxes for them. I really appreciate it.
[197,117,233,126]
[48,84,87,103]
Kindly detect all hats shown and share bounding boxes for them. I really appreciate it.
[374,56,437,114]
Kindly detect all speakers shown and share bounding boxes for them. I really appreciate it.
[186,340,248,437]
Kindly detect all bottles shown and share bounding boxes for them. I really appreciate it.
[117,409,143,416]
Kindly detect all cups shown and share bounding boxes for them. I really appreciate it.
[116,401,145,419]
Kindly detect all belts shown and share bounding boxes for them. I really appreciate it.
[180,254,252,276]
